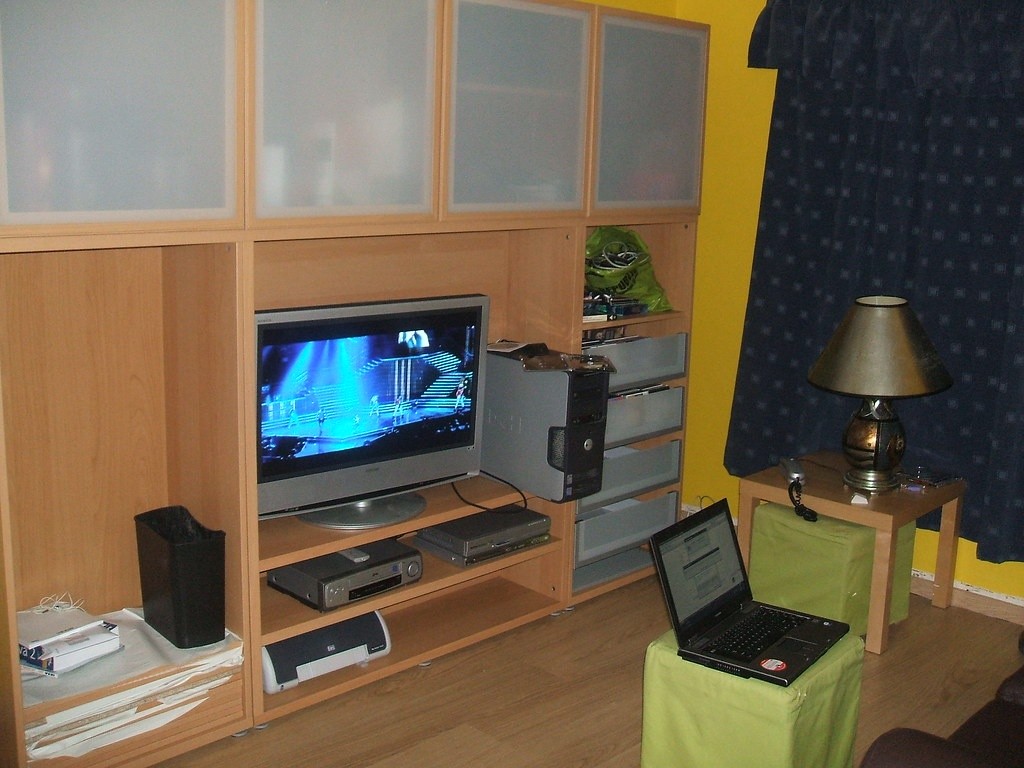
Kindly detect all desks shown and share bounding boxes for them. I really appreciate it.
[739,445,967,655]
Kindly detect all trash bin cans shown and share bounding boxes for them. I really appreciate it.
[129,504,233,649]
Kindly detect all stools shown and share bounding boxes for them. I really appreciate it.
[746,502,917,634]
[639,629,865,768]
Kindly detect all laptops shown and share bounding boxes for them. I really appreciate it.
[649,497,851,688]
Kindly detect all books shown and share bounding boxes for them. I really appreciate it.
[15,602,127,678]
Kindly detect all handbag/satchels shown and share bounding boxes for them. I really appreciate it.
[134,505,226,648]
[585,227,672,314]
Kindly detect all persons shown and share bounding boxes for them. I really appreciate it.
[287,377,468,435]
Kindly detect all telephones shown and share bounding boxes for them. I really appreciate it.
[779,455,806,487]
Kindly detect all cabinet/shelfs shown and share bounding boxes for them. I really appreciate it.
[0,0,713,768]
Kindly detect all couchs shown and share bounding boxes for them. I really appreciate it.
[859,630,1024,768]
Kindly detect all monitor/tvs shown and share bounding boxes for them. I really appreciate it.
[254,292,489,529]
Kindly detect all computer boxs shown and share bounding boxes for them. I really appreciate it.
[479,342,610,504]
